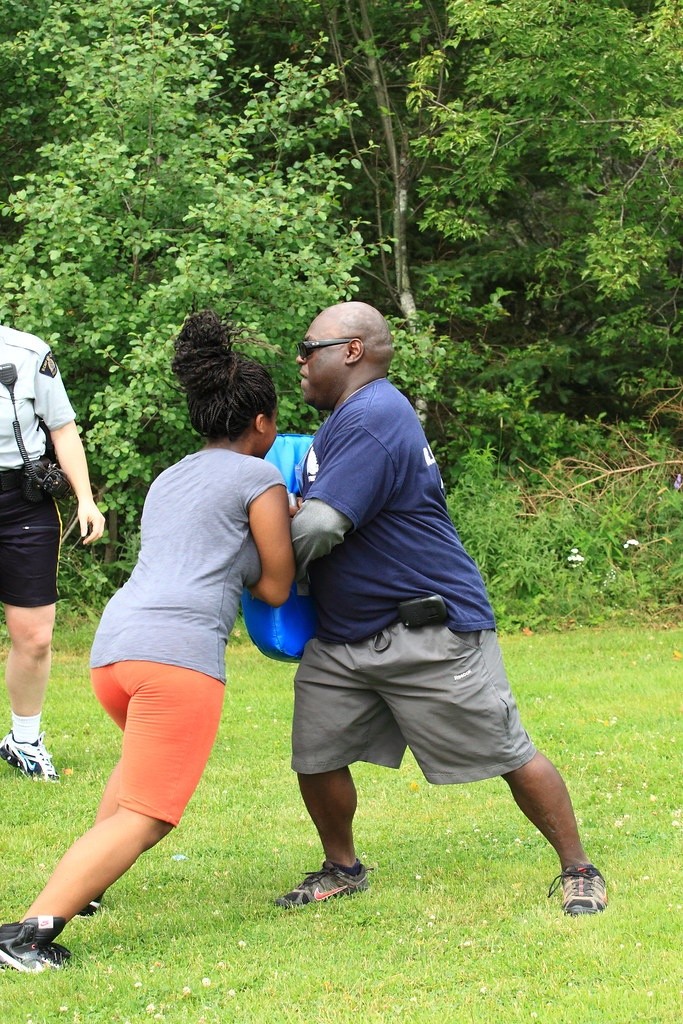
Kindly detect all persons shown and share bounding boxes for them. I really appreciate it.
[0,322,106,777]
[275,299,610,920]
[1,312,294,974]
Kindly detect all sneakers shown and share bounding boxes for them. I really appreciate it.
[272,857,374,908]
[560,861,608,915]
[0,915,74,978]
[1,727,62,784]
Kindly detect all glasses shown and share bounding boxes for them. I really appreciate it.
[293,336,358,363]
